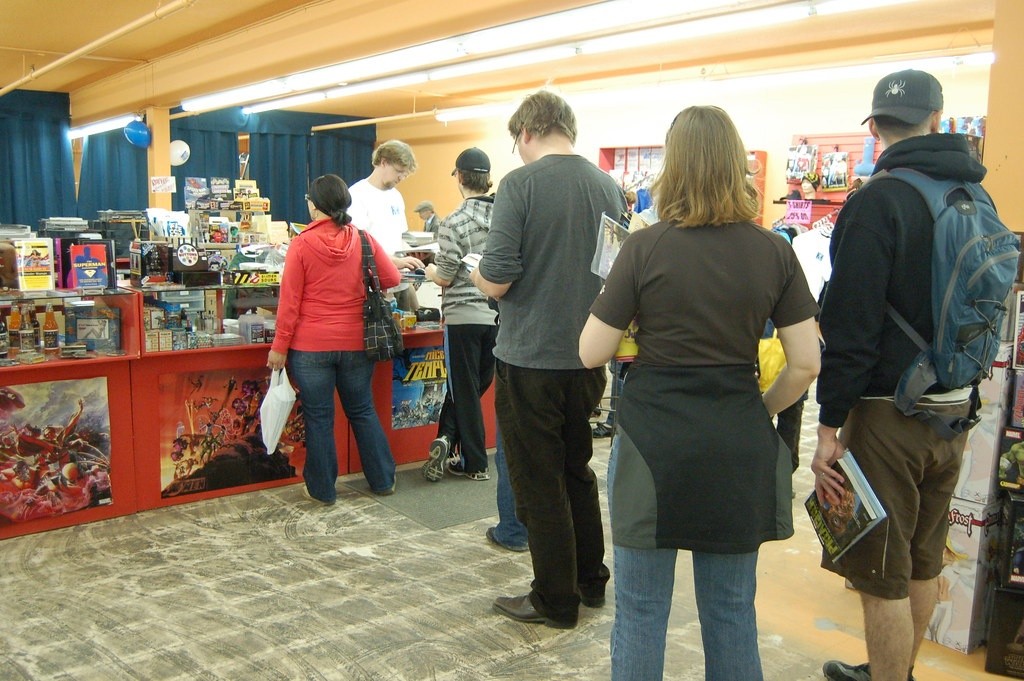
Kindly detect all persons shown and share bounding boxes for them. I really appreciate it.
[483,296,531,552]
[470,90,625,626]
[421,147,500,482]
[591,186,660,438]
[344,140,425,315]
[755,318,803,498]
[811,68,998,681]
[577,106,824,681]
[266,171,401,503]
[413,201,442,238]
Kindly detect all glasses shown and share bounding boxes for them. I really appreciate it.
[392,163,409,177]
[305,194,311,200]
[512,129,521,160]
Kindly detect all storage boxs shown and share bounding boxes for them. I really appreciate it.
[954,343,1015,506]
[925,499,1003,657]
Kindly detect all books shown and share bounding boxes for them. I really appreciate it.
[803,448,887,565]
[0,236,117,291]
[589,207,652,281]
[396,243,441,254]
[402,231,435,246]
[49,218,83,221]
[461,253,483,273]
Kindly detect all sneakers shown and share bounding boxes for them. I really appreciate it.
[422,435,451,482]
[822,660,914,681]
[448,461,490,480]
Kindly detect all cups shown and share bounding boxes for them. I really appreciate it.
[399,315,417,333]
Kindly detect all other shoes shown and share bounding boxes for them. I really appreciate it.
[378,474,396,494]
[593,423,613,438]
[487,527,528,551]
[302,484,335,506]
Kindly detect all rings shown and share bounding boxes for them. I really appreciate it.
[819,473,825,477]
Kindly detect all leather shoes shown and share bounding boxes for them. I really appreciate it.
[579,590,606,607]
[493,595,578,629]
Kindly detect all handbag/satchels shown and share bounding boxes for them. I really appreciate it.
[786,141,818,184]
[821,148,849,192]
[758,328,787,393]
[260,367,295,455]
[357,227,402,361]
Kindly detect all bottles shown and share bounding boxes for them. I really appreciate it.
[0,304,60,361]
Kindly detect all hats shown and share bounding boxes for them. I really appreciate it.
[860,69,942,124]
[414,202,434,211]
[802,172,820,192]
[451,147,490,176]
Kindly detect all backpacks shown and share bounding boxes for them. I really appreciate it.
[846,168,1018,416]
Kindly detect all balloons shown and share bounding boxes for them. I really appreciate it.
[124,120,150,148]
[171,139,190,166]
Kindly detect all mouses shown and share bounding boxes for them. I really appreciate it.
[414,268,426,275]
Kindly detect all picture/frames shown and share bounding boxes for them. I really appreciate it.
[63,306,121,353]
[78,238,118,291]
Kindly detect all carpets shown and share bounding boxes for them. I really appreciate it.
[340,453,607,532]
